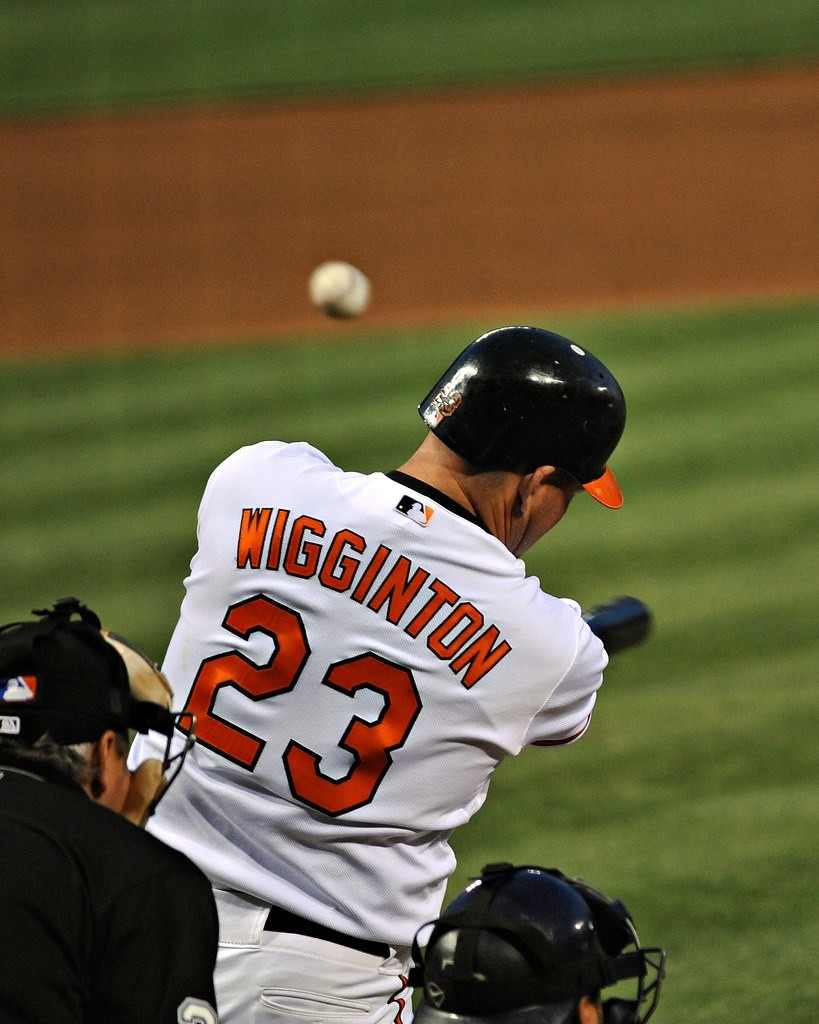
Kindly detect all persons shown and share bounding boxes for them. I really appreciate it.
[122,322,629,1024]
[0,595,220,1024]
[406,860,668,1024]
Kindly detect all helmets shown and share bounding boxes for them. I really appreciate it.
[418,326,623,509]
[411,865,607,1023]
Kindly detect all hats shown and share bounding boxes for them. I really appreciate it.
[0,618,169,743]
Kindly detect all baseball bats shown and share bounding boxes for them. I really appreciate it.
[579,591,656,657]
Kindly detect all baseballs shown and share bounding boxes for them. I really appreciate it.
[304,259,373,321]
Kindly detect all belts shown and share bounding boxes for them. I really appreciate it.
[262,905,390,959]
